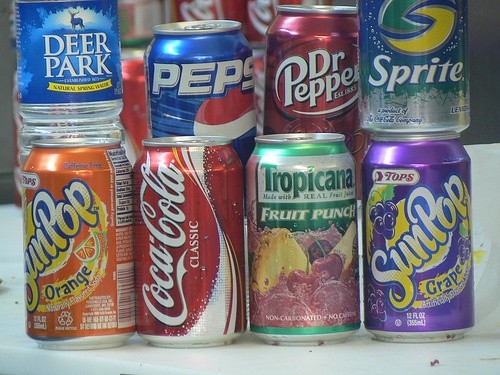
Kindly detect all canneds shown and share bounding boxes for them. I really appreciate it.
[12,0,476,350]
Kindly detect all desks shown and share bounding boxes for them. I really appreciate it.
[1,143,500,375]
